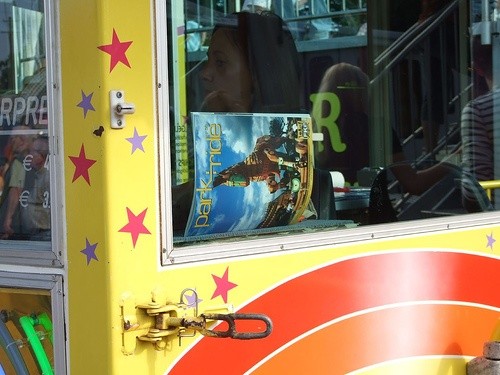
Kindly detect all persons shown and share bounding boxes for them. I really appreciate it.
[460,20,500,212]
[1,127,51,242]
[170,11,340,219]
[309,63,402,185]
